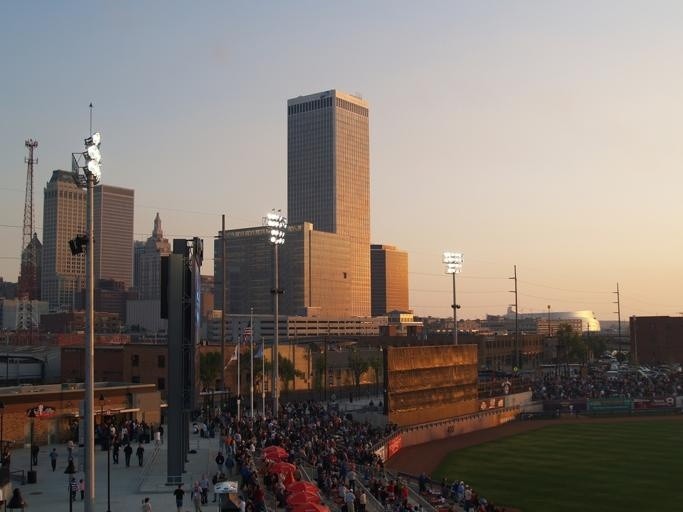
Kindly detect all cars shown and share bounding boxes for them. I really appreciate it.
[477,370,512,396]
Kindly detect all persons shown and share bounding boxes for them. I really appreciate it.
[172,484,185,511]
[140,497,153,512]
[94,416,163,467]
[48,447,57,472]
[32,442,39,465]
[67,477,78,501]
[531,374,682,402]
[78,478,84,498]
[416,472,506,512]
[66,438,75,456]
[188,399,423,512]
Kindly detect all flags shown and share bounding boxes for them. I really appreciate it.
[253,341,262,358]
[223,343,237,370]
[241,326,251,343]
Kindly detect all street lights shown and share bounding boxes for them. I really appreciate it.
[443,251,464,346]
[264,206,289,422]
[74,102,101,512]
